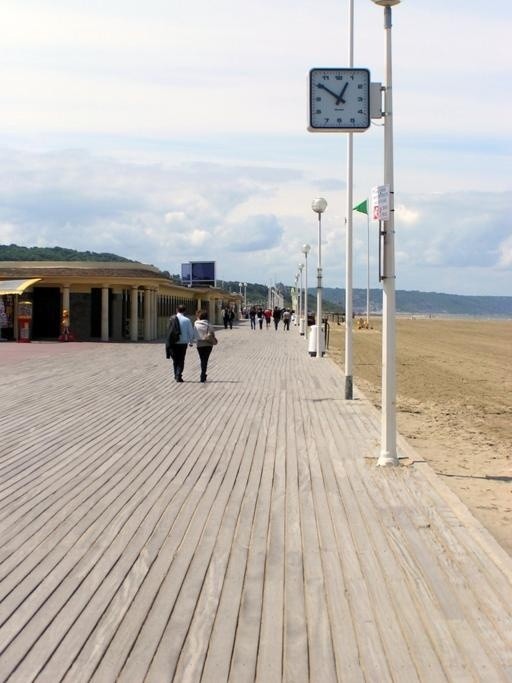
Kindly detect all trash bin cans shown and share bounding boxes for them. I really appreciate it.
[299,318,305,335]
[309,325,326,357]
[291,314,294,321]
[294,315,297,326]
[18,315,32,343]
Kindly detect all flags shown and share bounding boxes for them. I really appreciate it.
[353,199,367,214]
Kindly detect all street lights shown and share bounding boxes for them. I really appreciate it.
[239,241,311,339]
[311,196,327,358]
[373,0,403,469]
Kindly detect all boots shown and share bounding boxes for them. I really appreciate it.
[175,374,184,382]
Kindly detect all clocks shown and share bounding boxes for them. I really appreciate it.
[306,67,371,132]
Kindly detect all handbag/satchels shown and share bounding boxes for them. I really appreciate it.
[205,330,218,344]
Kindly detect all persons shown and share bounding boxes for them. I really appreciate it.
[221,304,312,331]
[165,304,193,382]
[191,311,216,383]
